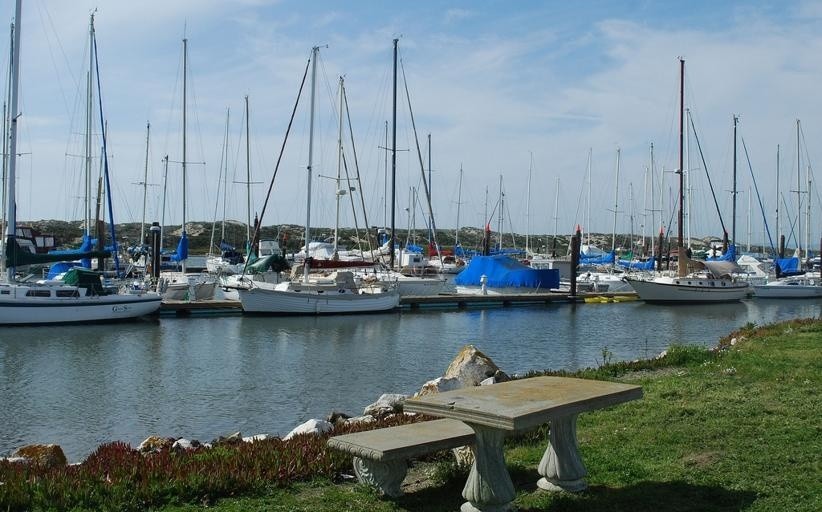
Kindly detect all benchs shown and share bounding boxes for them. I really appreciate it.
[324,418,474,498]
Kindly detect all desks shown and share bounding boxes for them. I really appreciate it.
[404,375,644,512]
